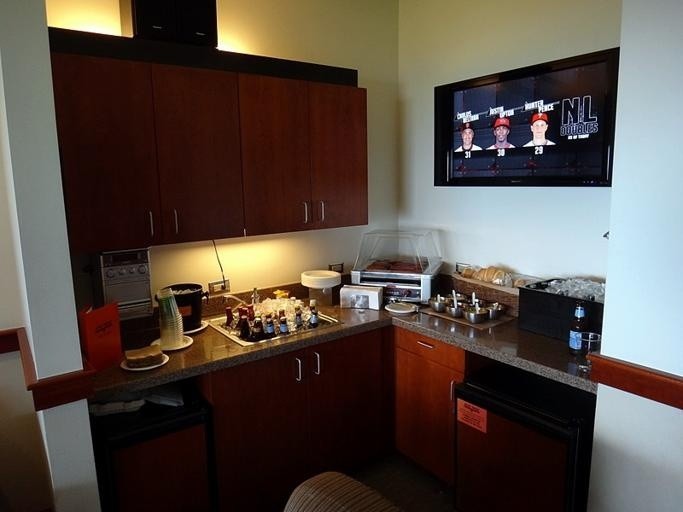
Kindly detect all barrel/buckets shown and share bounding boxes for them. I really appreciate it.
[160,284,209,332]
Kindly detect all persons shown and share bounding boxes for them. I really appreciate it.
[453,120,482,155]
[485,117,519,150]
[519,112,559,148]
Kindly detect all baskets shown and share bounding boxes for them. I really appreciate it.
[451,272,519,317]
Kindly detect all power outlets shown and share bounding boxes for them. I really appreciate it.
[208,279,230,296]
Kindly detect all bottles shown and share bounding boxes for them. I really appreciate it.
[223,298,318,342]
[569,300,587,354]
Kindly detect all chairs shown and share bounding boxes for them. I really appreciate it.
[285,471,399,512]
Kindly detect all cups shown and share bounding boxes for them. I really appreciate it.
[575,331,601,370]
[158,288,184,349]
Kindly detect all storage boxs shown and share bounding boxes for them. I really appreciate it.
[520,278,605,344]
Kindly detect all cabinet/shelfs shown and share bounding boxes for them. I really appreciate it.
[238,73,368,235]
[193,324,395,512]
[392,324,496,487]
[51,52,244,256]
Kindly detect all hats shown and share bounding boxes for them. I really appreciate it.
[494,118,509,129]
[461,124,474,132]
[532,113,548,124]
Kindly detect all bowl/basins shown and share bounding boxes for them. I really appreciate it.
[429,292,506,322]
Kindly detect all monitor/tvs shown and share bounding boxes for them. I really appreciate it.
[434,46,619,187]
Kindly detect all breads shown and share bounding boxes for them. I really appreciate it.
[464,265,529,288]
[125,344,164,369]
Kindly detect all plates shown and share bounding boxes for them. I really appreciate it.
[119,354,169,371]
[150,335,193,351]
[300,270,342,288]
[384,303,417,314]
[181,321,209,334]
